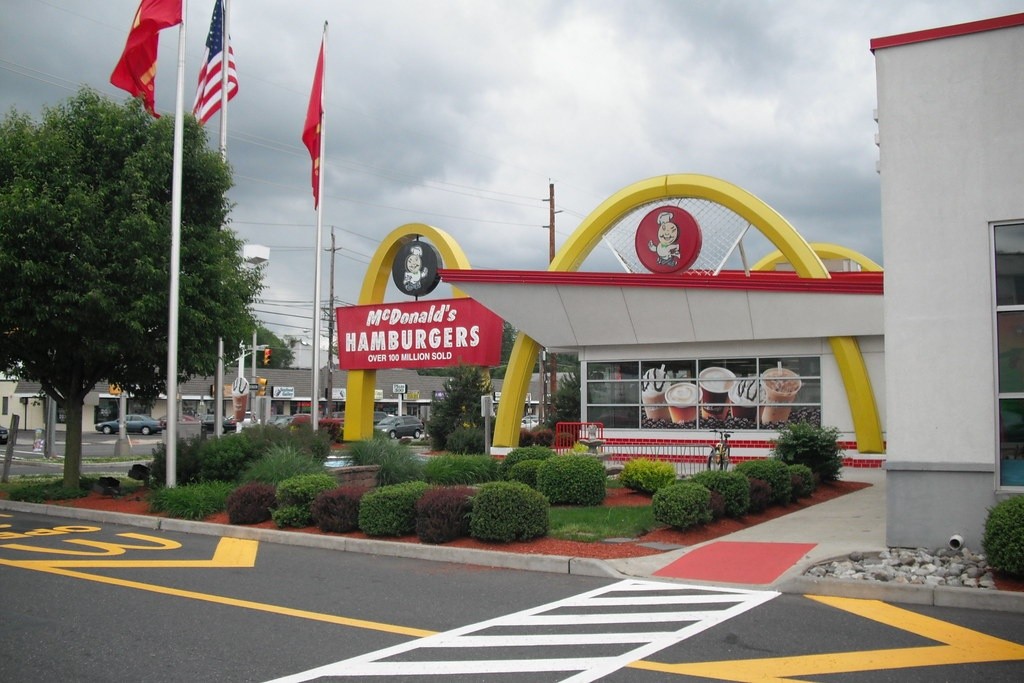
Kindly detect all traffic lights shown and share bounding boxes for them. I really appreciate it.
[264,348,272,365]
[257,378,268,396]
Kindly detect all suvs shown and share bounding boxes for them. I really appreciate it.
[374,415,423,440]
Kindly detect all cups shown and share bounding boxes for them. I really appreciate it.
[700,367,736,421]
[665,382,703,423]
[728,376,767,421]
[760,368,801,423]
[641,364,671,421]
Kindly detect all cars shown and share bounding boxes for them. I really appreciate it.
[0,426,8,443]
[243,412,261,428]
[322,411,344,426]
[375,411,386,422]
[95,414,163,435]
[268,414,311,427]
[158,414,203,430]
[196,414,237,434]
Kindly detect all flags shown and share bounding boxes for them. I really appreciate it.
[193,0,238,128]
[110,1,182,119]
[301,31,323,209]
